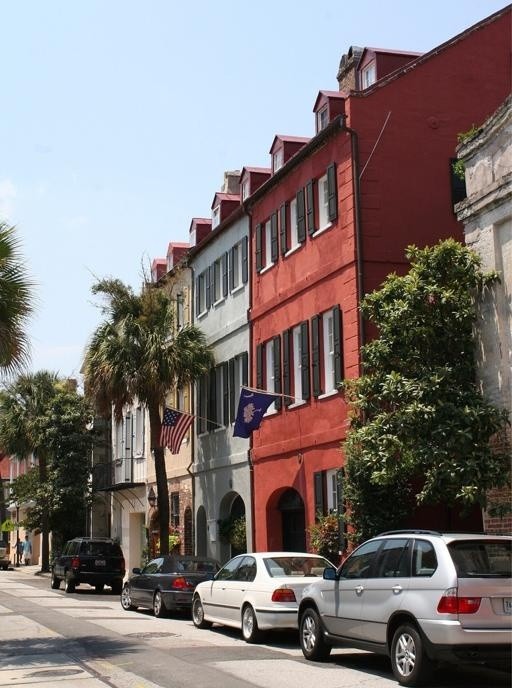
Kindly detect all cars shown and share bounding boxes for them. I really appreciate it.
[192,552,338,644]
[0,540,9,570]
[121,554,222,618]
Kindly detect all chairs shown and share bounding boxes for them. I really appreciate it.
[271,568,285,575]
[291,571,305,575]
[311,567,326,575]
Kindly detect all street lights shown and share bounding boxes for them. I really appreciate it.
[14,499,21,567]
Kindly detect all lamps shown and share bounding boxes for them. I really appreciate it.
[148,486,157,507]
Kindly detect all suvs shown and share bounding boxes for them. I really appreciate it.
[51,537,125,594]
[298,529,512,686]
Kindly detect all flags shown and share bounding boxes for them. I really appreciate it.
[158,407,195,455]
[232,386,279,439]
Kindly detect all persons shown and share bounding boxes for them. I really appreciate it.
[12,538,23,563]
[21,536,32,565]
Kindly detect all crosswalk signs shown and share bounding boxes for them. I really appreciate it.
[2,520,14,531]
